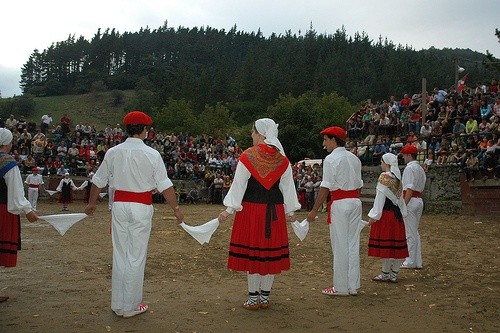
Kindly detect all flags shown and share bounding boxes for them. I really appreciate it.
[457,75,469,93]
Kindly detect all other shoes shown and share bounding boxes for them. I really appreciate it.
[259,300,270,309]
[241,301,259,311]
[371,274,391,282]
[399,262,423,269]
[321,287,349,296]
[62,207,68,211]
[116,304,148,318]
[389,271,399,283]
[349,289,358,296]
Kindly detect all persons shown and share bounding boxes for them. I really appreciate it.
[398,145,427,269]
[0,127,40,303]
[218,118,301,310]
[368,153,409,283]
[307,127,364,296]
[0,79,500,211]
[84,111,183,319]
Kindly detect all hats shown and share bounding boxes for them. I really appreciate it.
[255,118,286,157]
[320,126,347,141]
[89,172,94,176]
[0,128,13,145]
[123,111,153,125]
[400,146,418,154]
[382,153,402,180]
[32,167,39,171]
[65,173,69,176]
